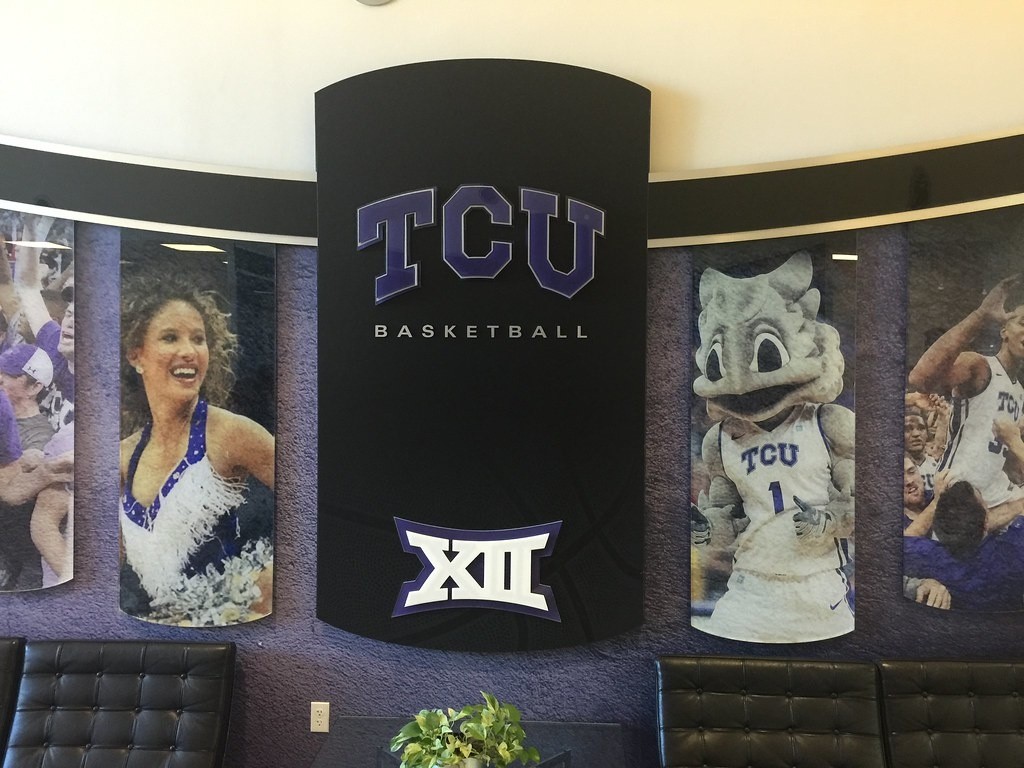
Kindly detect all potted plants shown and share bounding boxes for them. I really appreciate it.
[390,692,540,768]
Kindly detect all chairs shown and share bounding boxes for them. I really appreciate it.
[654,654,888,768]
[2,639,235,768]
[876,659,1024,768]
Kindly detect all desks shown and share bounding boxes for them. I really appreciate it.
[307,715,625,768]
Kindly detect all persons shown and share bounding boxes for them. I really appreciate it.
[0,207,74,593]
[905,272,1024,614]
[120,274,274,629]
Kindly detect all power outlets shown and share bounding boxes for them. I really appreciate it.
[310,701,329,732]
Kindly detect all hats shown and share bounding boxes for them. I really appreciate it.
[0,343,54,391]
